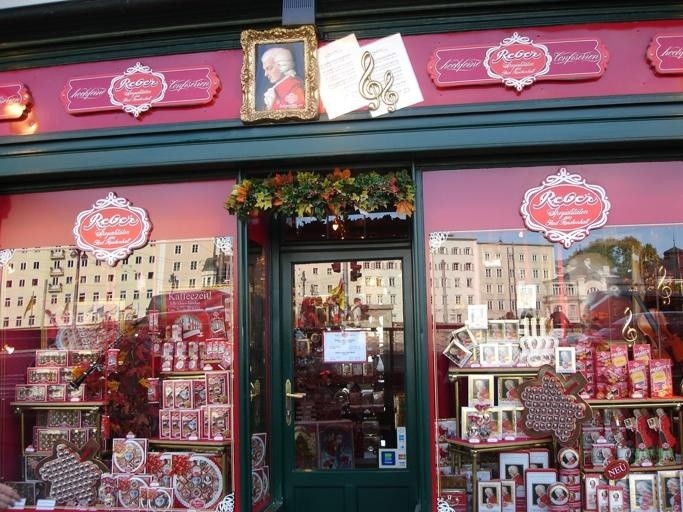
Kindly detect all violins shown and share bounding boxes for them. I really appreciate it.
[623,279,682,365]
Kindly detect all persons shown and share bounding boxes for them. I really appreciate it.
[508,465,523,485]
[475,380,488,401]
[633,409,651,449]
[636,481,652,508]
[667,477,681,509]
[501,486,511,502]
[534,485,551,506]
[652,408,676,447]
[565,252,634,335]
[0,482,21,510]
[483,488,496,504]
[348,298,362,321]
[260,47,305,111]
[505,379,519,400]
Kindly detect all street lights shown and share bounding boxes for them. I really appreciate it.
[70,248,88,326]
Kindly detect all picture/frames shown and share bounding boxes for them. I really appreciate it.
[443,303,577,440]
[240,24,320,124]
[477,449,682,512]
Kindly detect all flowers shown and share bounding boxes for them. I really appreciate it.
[221,164,419,228]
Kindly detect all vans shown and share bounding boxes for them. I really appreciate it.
[584,290,683,396]
